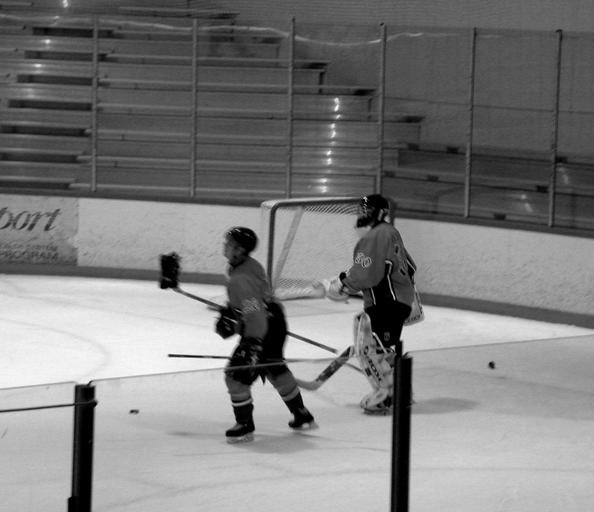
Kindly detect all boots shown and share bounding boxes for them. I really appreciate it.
[225,405,255,437]
[284,392,313,427]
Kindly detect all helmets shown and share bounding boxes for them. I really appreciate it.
[226,227,257,254]
[354,195,389,228]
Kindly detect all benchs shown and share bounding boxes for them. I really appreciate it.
[0,0,594,237]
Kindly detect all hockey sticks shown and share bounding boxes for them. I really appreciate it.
[291,346,355,390]
[159,253,341,355]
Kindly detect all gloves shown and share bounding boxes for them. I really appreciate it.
[214,310,238,338]
[239,338,265,366]
[320,271,350,302]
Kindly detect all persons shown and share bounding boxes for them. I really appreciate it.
[320,194,417,413]
[215,227,318,441]
